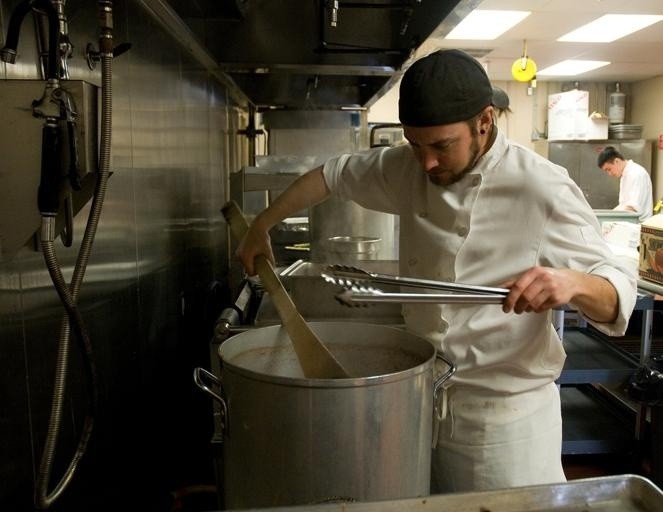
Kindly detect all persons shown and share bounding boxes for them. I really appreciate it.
[597,146,652,222]
[234,46,641,491]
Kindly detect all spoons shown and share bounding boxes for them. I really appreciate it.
[218,199,351,380]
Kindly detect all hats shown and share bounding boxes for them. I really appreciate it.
[398,47,511,128]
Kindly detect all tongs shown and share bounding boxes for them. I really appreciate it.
[320,264,513,309]
